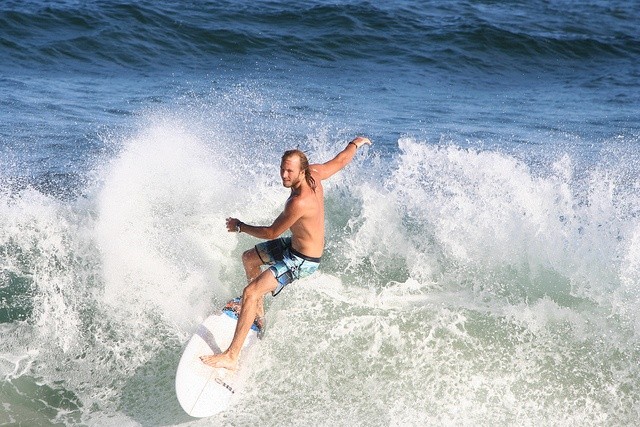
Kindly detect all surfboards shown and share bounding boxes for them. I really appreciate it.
[175,293,260,418]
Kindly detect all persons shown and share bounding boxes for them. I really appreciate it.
[199,136,371,370]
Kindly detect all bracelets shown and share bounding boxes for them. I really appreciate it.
[349,142,357,149]
[236,222,243,233]
